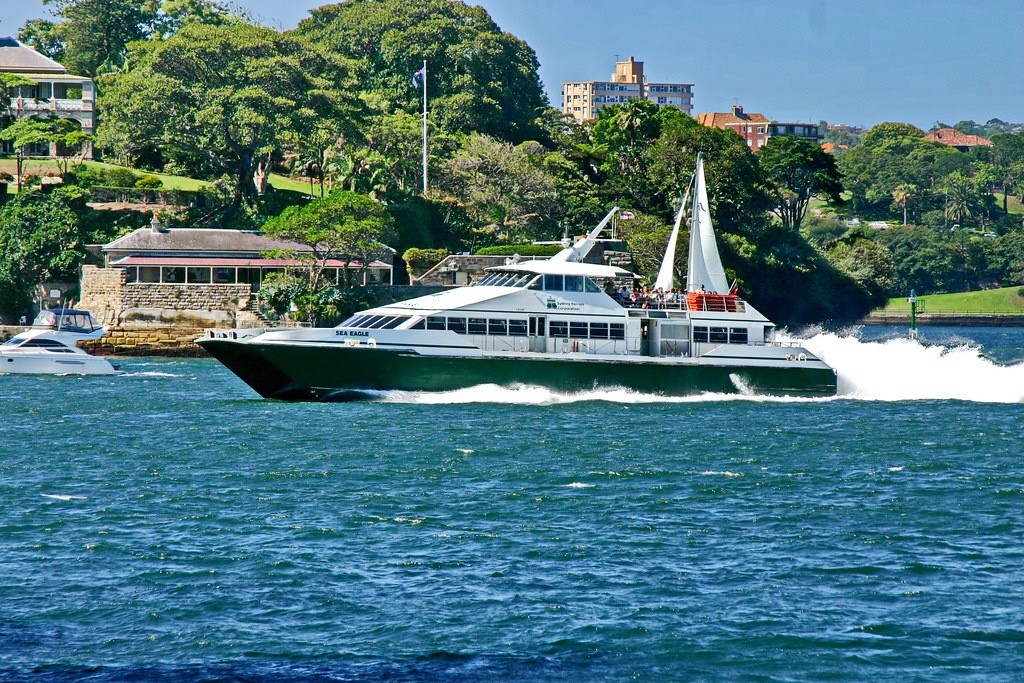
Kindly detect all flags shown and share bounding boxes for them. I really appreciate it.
[412,67,424,88]
[620,211,634,220]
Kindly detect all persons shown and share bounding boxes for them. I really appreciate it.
[621,283,717,311]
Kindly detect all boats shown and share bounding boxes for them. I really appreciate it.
[30,309,103,345]
[194,152,837,399]
[0,329,126,375]
